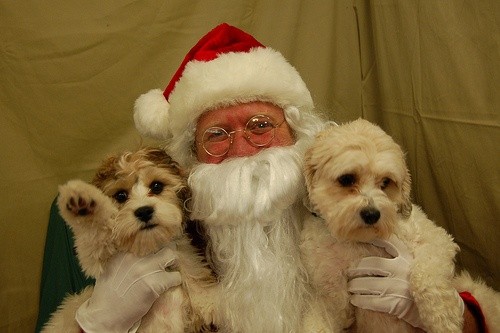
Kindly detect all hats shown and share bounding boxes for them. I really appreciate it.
[133,22,315,140]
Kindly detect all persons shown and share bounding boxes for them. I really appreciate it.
[40,23,500,333]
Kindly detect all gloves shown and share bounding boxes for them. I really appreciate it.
[75,246,182,333]
[346,228,428,331]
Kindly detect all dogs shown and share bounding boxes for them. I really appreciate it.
[300,118,465,333]
[55,144,228,333]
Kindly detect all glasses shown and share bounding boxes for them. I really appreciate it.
[196,114,285,157]
